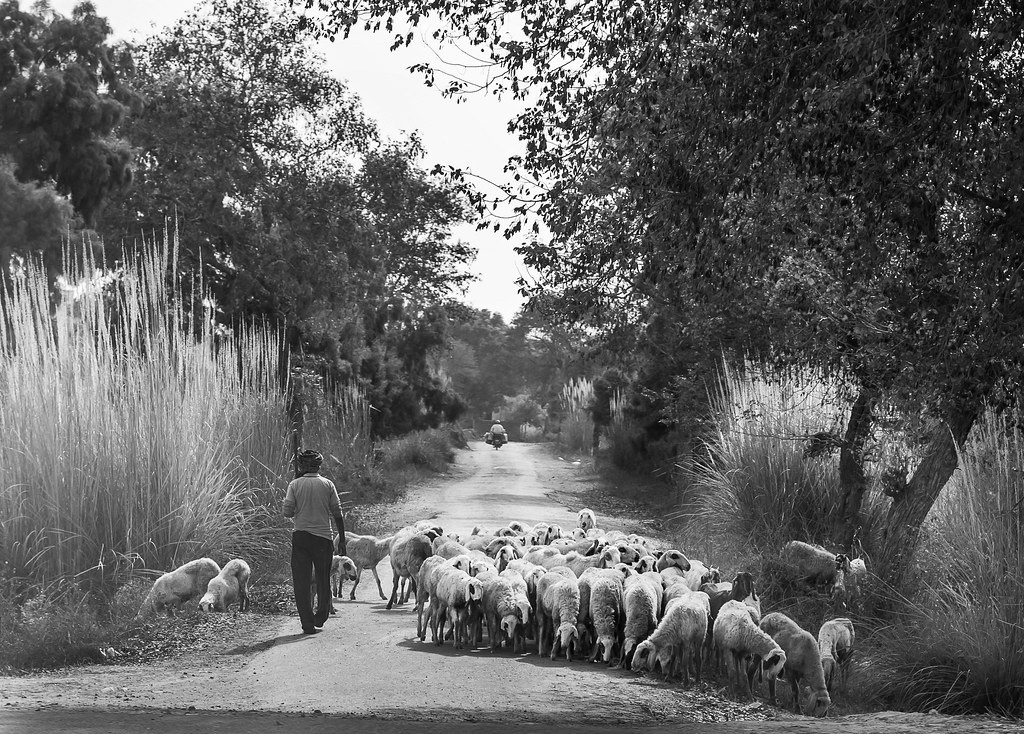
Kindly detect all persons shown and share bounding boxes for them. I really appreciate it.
[490,420,505,448]
[282,449,347,639]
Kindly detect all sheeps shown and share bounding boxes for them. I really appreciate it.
[199,559,251,616]
[131,556,221,622]
[330,508,868,716]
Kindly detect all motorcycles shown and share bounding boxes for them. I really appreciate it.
[491,434,503,450]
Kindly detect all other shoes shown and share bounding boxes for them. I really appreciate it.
[305,628,315,634]
[314,614,323,628]
